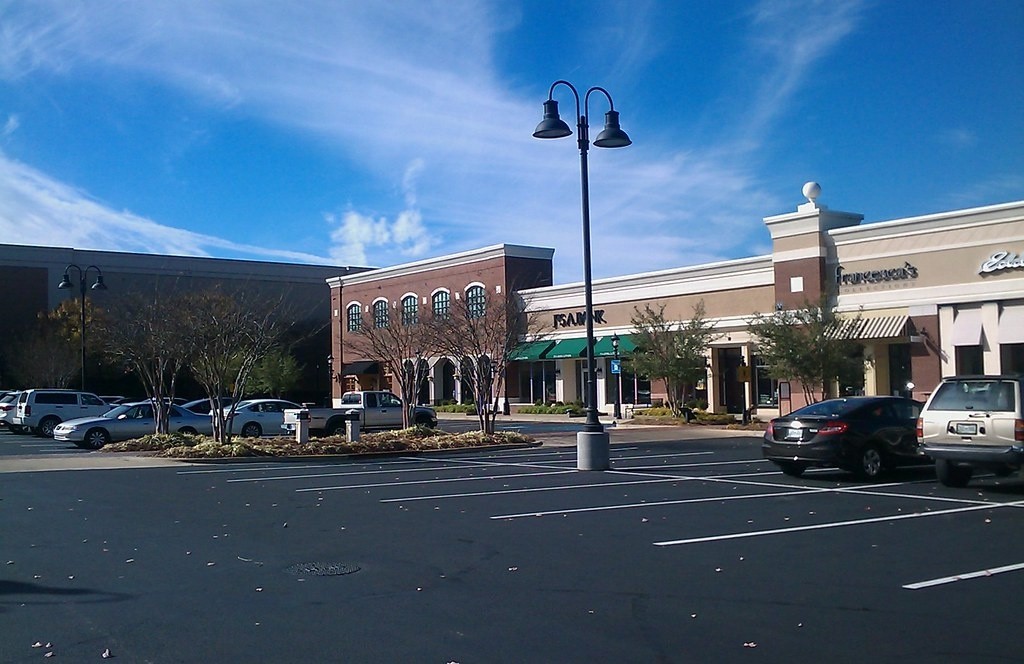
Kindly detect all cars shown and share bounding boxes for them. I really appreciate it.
[916,376,1024,488]
[762,396,924,479]
[208,399,306,438]
[0,388,237,450]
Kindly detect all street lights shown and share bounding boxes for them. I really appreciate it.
[611,334,620,417]
[58,263,108,392]
[327,354,334,407]
[532,80,632,471]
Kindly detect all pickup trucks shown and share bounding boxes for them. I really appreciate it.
[281,391,438,438]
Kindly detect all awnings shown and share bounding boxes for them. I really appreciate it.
[996,304,1023,343]
[508,340,555,362]
[546,337,597,359]
[593,333,646,357]
[949,305,985,349]
[815,316,910,342]
